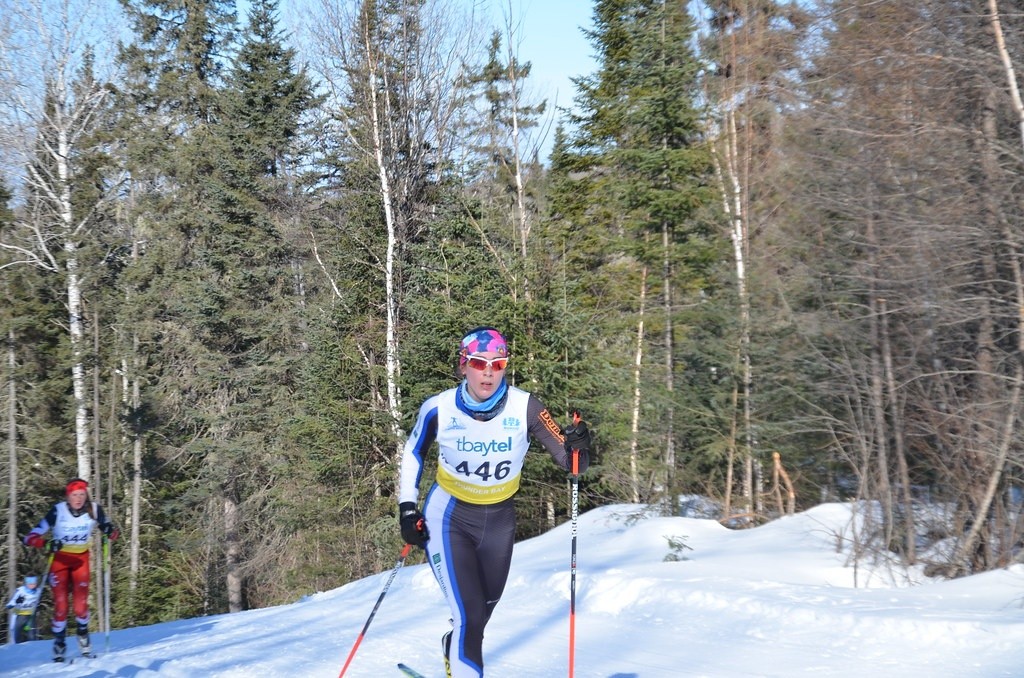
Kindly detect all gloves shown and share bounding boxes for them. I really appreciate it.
[98,521,115,536]
[44,539,63,552]
[559,420,590,454]
[399,502,430,546]
[16,595,24,603]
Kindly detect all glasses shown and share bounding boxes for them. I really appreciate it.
[459,352,508,371]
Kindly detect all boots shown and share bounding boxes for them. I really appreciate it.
[77,614,91,654]
[51,619,67,662]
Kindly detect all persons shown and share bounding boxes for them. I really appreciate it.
[398,326,593,678]
[23,478,120,662]
[6,571,39,644]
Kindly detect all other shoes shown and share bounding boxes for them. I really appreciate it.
[441,630,452,678]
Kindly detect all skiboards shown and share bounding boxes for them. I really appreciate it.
[398,663,425,678]
[57,654,97,666]
